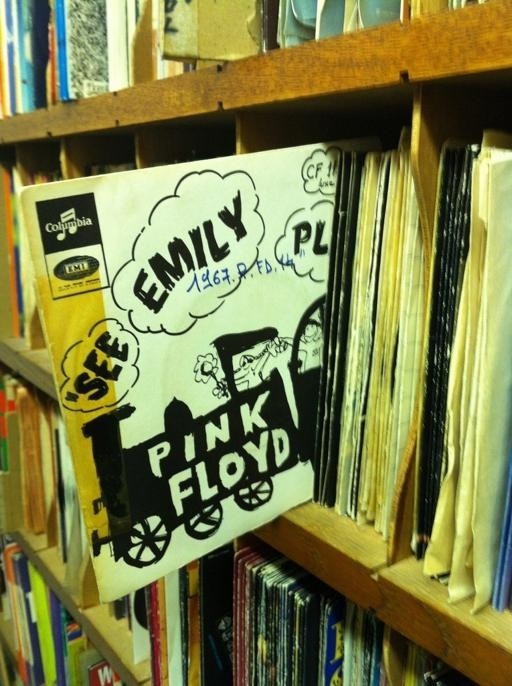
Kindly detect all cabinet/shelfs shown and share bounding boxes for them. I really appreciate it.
[1,0,512,686]
